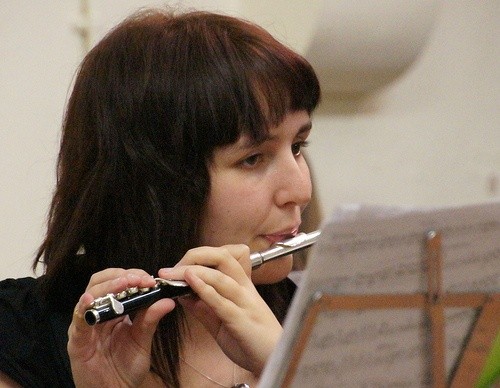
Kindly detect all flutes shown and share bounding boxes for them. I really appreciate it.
[84,230,323,325]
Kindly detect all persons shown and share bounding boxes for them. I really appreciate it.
[2,11,319,388]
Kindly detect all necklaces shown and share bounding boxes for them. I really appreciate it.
[180,354,250,388]
